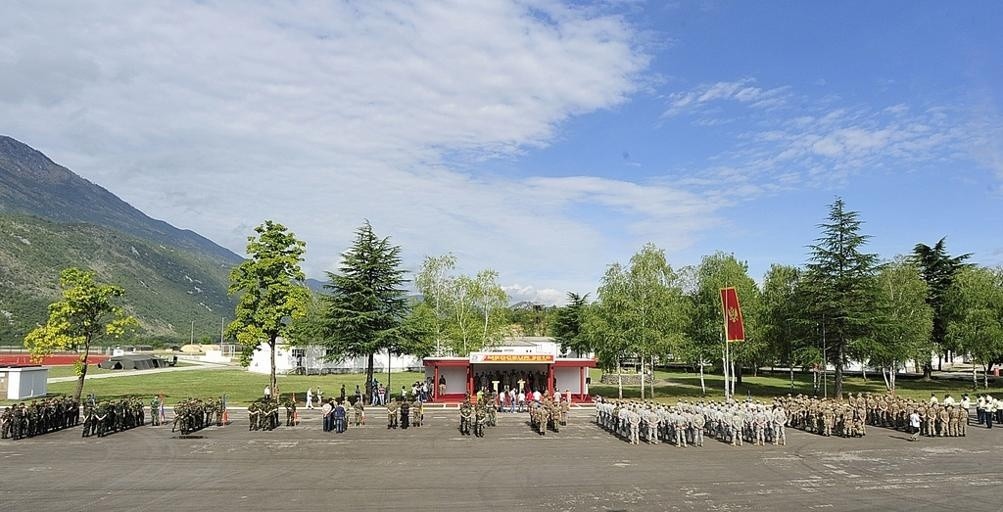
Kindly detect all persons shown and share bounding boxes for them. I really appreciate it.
[371,377,391,407]
[595,394,788,449]
[151,394,165,426]
[387,375,446,429]
[285,398,296,426]
[353,384,365,426]
[263,384,272,399]
[865,391,971,440]
[459,370,571,437]
[341,384,345,398]
[306,385,324,410]
[976,393,1003,429]
[172,396,225,435]
[248,397,278,431]
[786,392,866,438]
[82,393,145,438]
[275,385,281,405]
[1,394,81,440]
[320,396,351,433]
